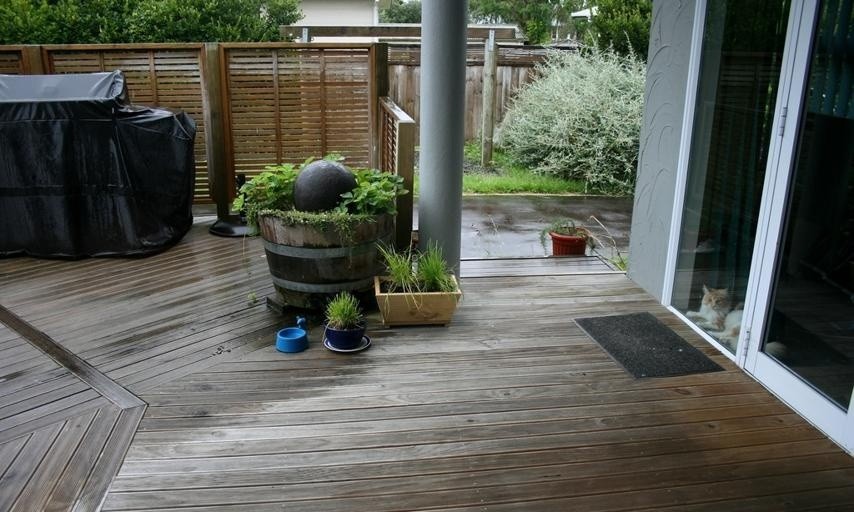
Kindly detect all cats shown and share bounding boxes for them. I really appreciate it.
[685,282,789,358]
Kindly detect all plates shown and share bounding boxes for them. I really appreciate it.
[323,335,372,353]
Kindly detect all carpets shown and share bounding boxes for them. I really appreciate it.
[768,309,853,366]
[571,311,728,380]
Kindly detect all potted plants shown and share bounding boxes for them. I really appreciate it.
[231,150,411,309]
[538,217,598,256]
[372,238,464,326]
[322,289,372,353]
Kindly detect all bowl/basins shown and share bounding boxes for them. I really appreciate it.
[275,327,309,353]
